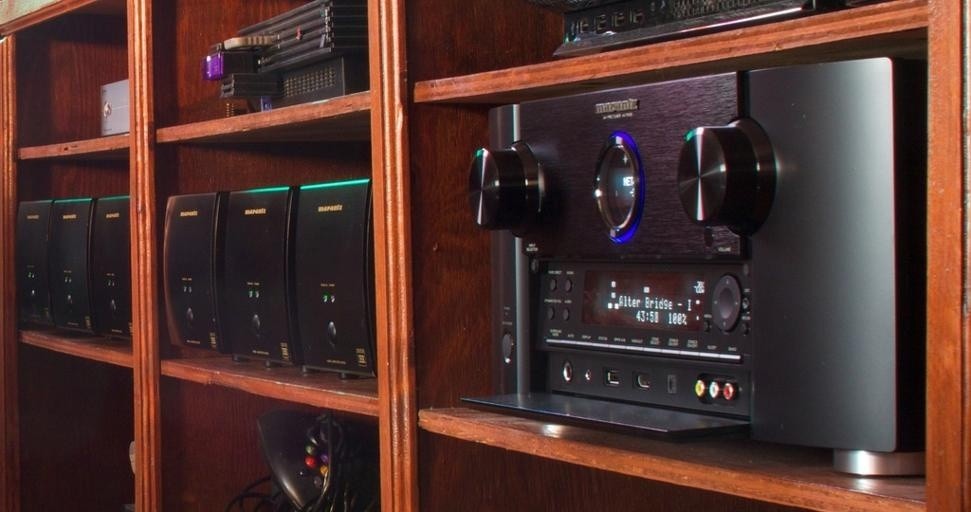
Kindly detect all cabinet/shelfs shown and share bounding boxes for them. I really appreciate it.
[0,1,971,511]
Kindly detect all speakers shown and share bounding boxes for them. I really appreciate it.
[15,198,53,324]
[222,187,297,367]
[100,77,131,136]
[91,195,131,338]
[52,198,95,335]
[162,193,225,352]
[296,179,376,380]
